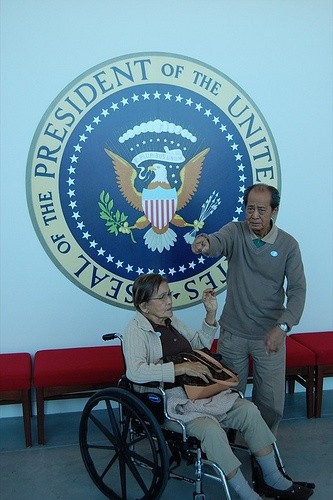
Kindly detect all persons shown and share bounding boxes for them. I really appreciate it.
[191,183,307,437]
[123,273,314,500]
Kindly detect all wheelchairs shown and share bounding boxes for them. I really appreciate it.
[79,333,317,500]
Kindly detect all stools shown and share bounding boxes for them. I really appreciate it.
[0,330,333,449]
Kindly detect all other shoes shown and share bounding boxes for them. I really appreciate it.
[262,481,314,500]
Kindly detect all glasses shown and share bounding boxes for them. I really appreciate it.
[146,290,174,302]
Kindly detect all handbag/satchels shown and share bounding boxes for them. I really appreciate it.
[164,347,238,400]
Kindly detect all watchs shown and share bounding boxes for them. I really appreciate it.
[279,322,289,332]
